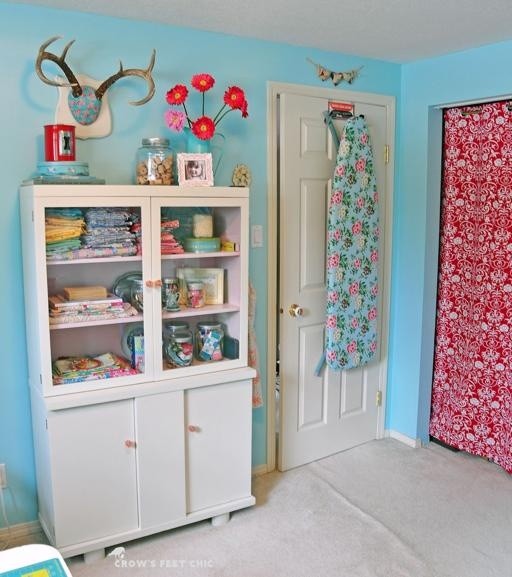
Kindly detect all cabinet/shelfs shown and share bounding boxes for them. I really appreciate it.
[20,181,259,565]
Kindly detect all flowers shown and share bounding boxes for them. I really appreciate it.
[163,73,250,140]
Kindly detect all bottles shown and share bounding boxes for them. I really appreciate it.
[164,322,193,367]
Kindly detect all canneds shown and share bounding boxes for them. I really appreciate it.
[164,323,193,368]
[44,125,75,161]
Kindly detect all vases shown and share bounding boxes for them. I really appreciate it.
[181,125,226,176]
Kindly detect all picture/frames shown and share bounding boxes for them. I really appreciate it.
[176,266,225,308]
[176,151,215,187]
